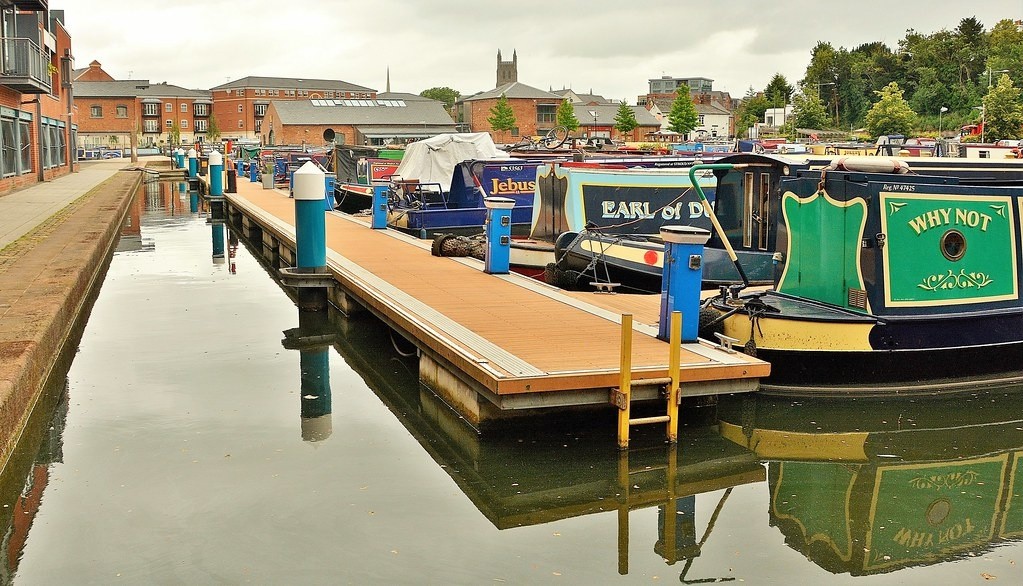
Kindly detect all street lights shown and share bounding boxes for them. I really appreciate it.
[989,67,1010,92]
[939,107,949,138]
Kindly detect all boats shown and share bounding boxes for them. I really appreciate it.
[711,382,1023,576]
[195,136,1023,396]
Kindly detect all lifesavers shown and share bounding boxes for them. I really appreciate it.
[829,156,911,175]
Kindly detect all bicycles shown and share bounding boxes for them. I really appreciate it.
[504,124,568,150]
